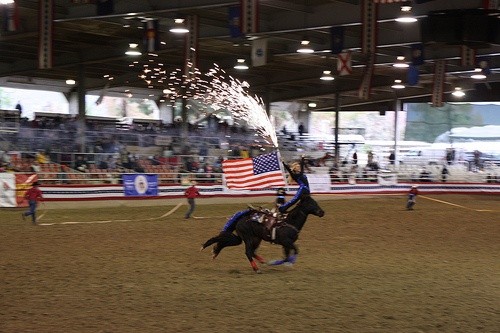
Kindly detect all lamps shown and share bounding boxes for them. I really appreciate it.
[296,41,314,53]
[471,68,486,80]
[393,56,410,68]
[125,43,143,56]
[391,80,405,89]
[320,71,335,80]
[169,13,190,34]
[395,6,417,22]
[233,59,249,69]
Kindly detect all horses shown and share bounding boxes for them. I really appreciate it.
[199,191,325,272]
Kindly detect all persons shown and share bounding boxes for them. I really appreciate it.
[184,181,201,219]
[405,186,419,210]
[275,186,286,207]
[0,98,500,190]
[266,160,310,268]
[22,181,45,225]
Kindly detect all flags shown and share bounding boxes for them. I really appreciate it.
[221,151,288,189]
